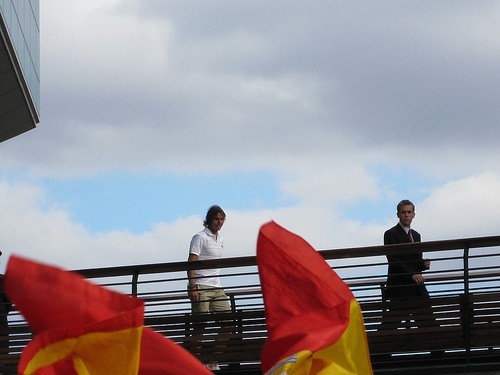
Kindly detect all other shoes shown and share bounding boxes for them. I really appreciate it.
[206,364,221,371]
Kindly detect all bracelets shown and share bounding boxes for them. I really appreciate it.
[189,284,197,289]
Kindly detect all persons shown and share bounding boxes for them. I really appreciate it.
[374,199,447,357]
[187,205,234,371]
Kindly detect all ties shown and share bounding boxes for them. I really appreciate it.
[406,229,414,245]
